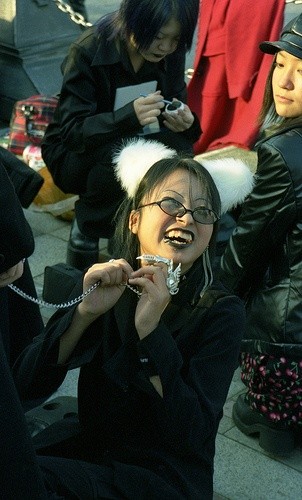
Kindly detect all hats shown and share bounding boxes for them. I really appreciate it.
[258,12,302,59]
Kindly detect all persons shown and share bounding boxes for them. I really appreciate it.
[39,0,203,273]
[210,14,302,458]
[0,148,44,369]
[10,137,257,500]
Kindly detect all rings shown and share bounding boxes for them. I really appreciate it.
[136,255,182,295]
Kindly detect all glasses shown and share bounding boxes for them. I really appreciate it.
[139,198,221,224]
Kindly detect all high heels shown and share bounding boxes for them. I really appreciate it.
[67,210,99,272]
[233,394,296,457]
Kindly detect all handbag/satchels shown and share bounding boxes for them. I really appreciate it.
[7,94,60,154]
[42,262,82,305]
